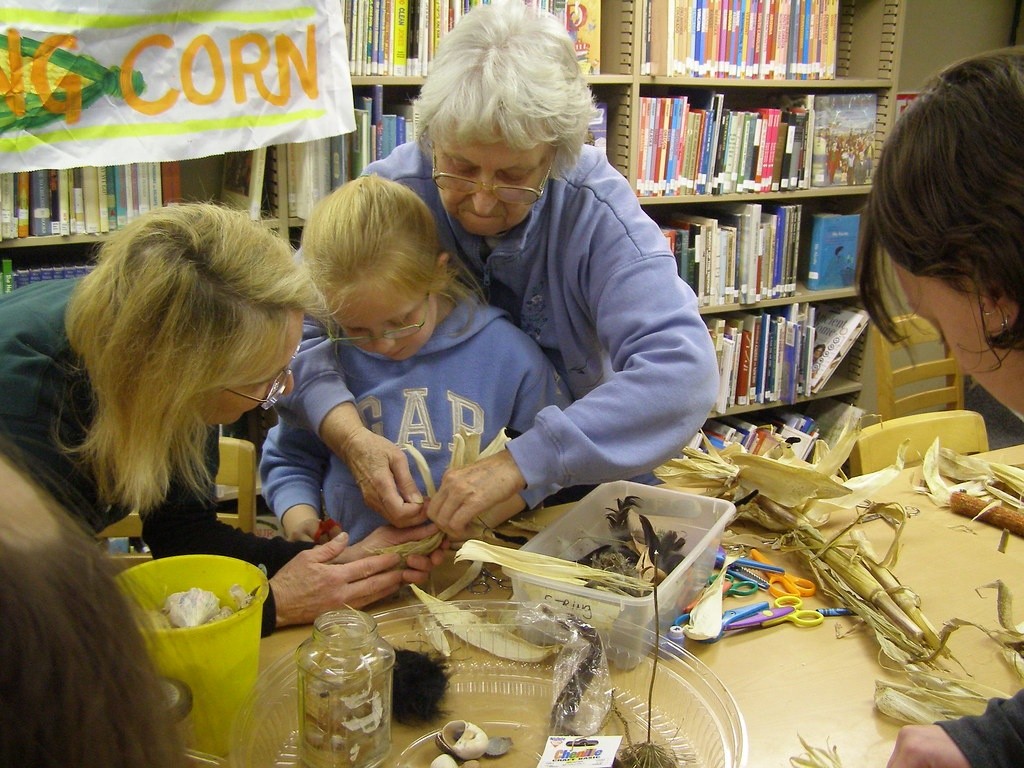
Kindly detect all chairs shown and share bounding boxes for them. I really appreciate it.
[213,432,268,529]
[846,403,991,481]
[868,312,969,423]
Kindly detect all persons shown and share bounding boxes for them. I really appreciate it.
[258,173,573,542]
[852,47,1024,768]
[0,202,448,639]
[283,0,721,539]
[0,454,185,768]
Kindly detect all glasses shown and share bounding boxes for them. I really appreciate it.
[432,142,558,205]
[327,292,429,345]
[222,344,301,410]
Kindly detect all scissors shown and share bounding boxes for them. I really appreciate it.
[699,572,760,603]
[722,595,823,633]
[750,545,816,600]
[673,603,771,641]
[467,559,513,595]
[717,548,785,590]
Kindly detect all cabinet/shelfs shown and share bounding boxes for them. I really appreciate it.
[0,0,911,536]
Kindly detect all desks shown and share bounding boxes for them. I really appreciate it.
[186,440,1024,768]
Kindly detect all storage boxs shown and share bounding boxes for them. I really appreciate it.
[505,472,749,671]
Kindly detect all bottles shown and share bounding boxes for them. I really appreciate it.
[296,609,395,768]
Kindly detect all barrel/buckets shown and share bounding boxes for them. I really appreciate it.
[114,553,269,761]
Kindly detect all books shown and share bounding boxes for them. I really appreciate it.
[220,0,876,460]
[0,161,182,296]
